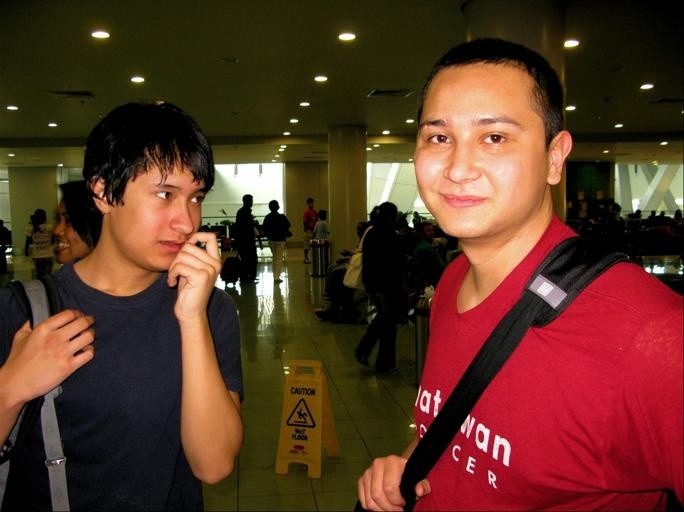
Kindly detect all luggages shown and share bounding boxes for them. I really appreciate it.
[219,252,242,285]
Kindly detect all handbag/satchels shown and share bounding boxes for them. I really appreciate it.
[341,224,373,291]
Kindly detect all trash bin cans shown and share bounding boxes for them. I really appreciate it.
[221,224,232,251]
[311,239,328,278]
[415,297,433,388]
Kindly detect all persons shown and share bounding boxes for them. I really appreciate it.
[0,99,246,511]
[50,179,102,266]
[312,196,458,377]
[356,33,684,512]
[611,200,684,274]
[302,196,320,263]
[262,200,291,287]
[234,193,262,286]
[21,206,55,278]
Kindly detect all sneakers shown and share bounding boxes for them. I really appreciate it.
[273,278,282,283]
[303,256,310,264]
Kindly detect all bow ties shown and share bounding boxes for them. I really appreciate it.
[354,352,368,368]
